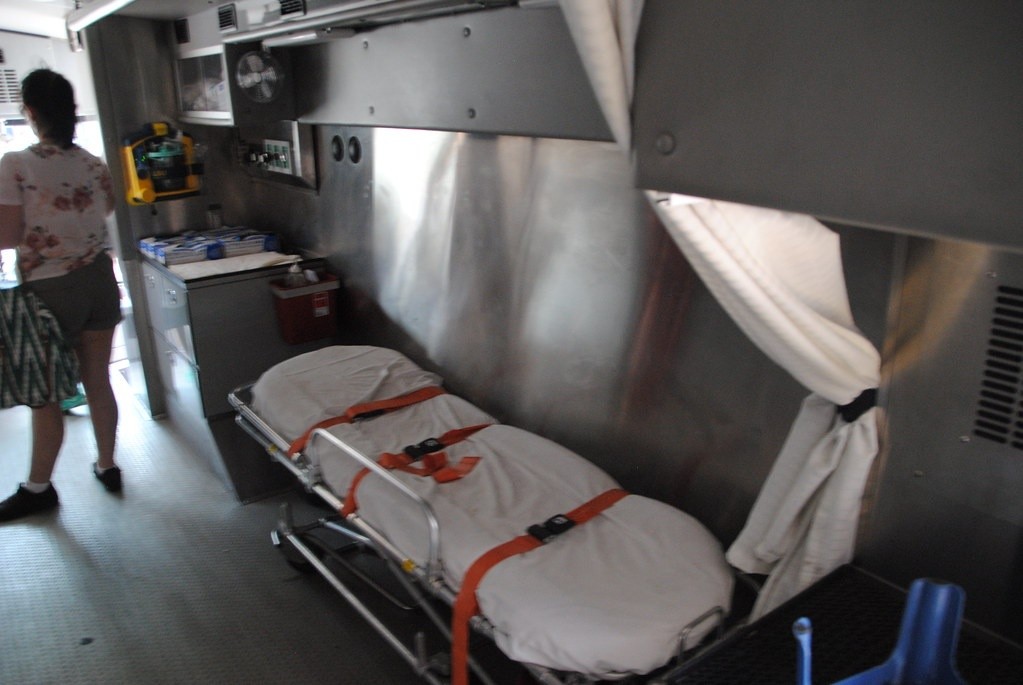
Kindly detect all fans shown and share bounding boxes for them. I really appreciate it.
[237,49,285,104]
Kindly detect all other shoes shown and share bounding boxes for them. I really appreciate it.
[0,482,58,522]
[94,462,122,492]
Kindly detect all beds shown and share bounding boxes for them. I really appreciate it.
[228,345,762,685]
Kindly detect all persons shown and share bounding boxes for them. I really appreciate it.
[0,70,124,523]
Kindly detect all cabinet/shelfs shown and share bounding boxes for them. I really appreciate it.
[142,262,342,504]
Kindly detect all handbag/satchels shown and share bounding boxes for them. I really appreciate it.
[0,247,81,410]
